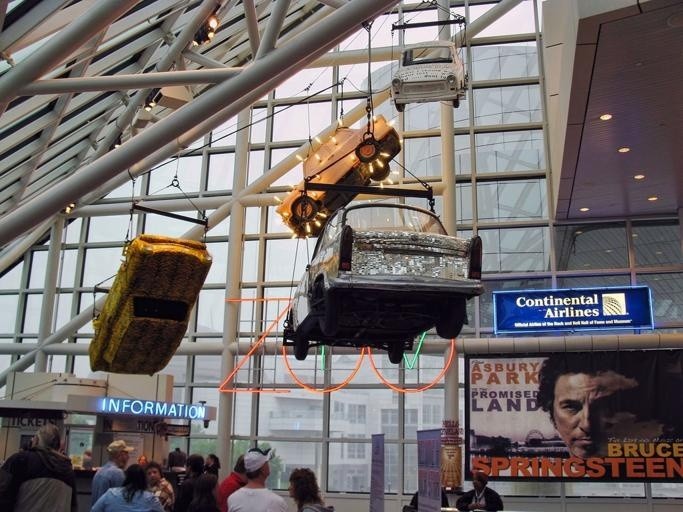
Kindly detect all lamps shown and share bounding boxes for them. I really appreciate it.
[388,119,395,126]
[283,211,288,219]
[375,159,383,168]
[336,118,345,129]
[380,152,390,158]
[331,137,338,146]
[369,162,374,174]
[385,178,392,184]
[379,181,383,189]
[349,153,354,161]
[313,218,320,227]
[372,115,377,122]
[295,155,305,164]
[288,183,294,190]
[314,154,322,162]
[272,197,281,202]
[314,138,323,145]
[306,221,312,235]
[290,233,296,241]
[317,211,327,218]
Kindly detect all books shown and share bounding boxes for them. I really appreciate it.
[441,508,458,512]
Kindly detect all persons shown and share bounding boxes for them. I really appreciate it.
[145,462,178,512]
[137,455,148,467]
[91,440,135,506]
[216,454,248,512]
[456,472,504,512]
[536,353,639,468]
[289,468,335,512]
[0,424,77,512]
[226,447,289,511]
[90,464,165,512]
[82,448,93,469]
[174,455,212,512]
[204,454,221,479]
[409,488,449,510]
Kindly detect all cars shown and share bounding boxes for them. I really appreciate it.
[282,199,485,364]
[391,40,466,113]
[275,113,402,240]
[88,234,213,378]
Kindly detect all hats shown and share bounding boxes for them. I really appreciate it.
[249,448,271,456]
[244,451,273,473]
[107,440,134,455]
